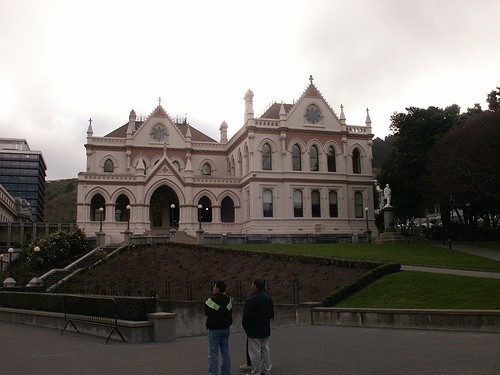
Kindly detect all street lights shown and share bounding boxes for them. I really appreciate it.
[0,254,3,270]
[28,246,42,286]
[195,204,205,244]
[365,207,372,243]
[95,207,106,246]
[168,203,177,242]
[6,248,18,287]
[120,205,133,242]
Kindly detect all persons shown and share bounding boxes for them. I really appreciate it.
[374,182,385,208]
[204,280,233,375]
[241,278,274,375]
[383,184,393,207]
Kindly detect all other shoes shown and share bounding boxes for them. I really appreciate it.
[239,363,251,372]
